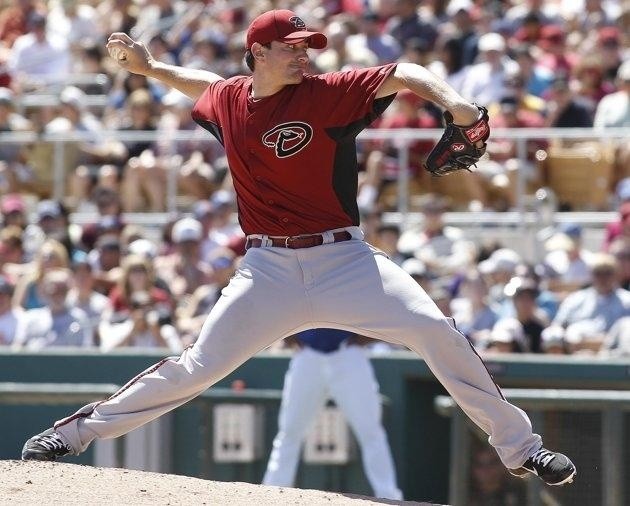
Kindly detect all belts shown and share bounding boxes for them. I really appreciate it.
[246,232,361,249]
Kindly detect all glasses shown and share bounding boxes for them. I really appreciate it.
[595,270,616,280]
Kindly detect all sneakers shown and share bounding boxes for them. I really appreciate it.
[21,424,74,461]
[506,446,577,484]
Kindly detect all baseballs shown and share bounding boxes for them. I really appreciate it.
[107,47,127,60]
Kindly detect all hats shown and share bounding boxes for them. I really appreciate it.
[5,214,243,342]
[330,5,618,94]
[393,219,617,300]
[243,9,327,49]
[5,5,241,118]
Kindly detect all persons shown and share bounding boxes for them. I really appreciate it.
[22,9,577,487]
[261,329,405,500]
[0,0,630,355]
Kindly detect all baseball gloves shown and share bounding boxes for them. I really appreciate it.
[423,103,490,177]
